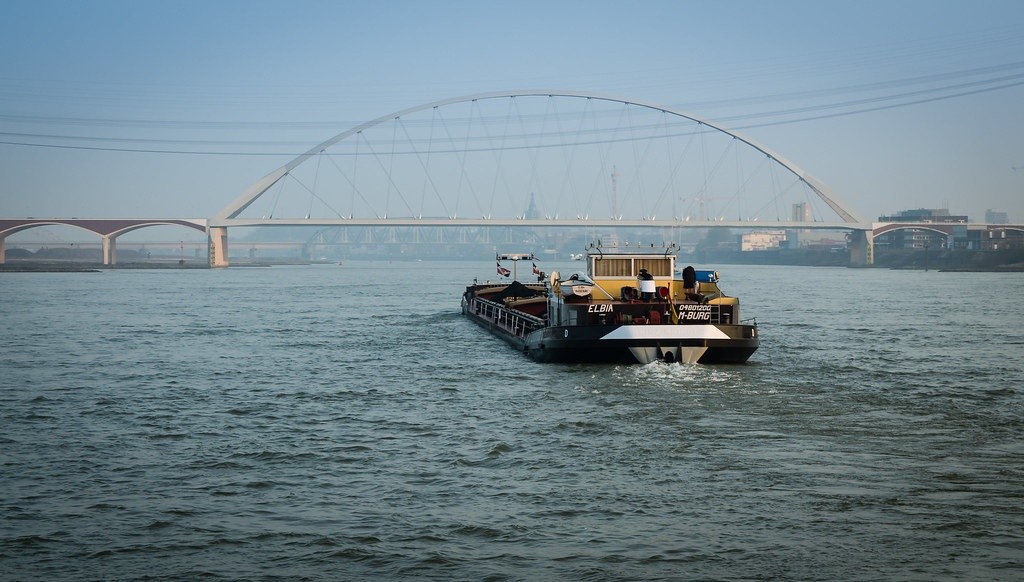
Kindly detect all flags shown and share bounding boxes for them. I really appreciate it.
[533,263,540,275]
[496,262,511,277]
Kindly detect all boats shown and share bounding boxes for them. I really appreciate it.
[460,240,762,366]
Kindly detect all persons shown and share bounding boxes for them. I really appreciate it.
[683,266,697,301]
